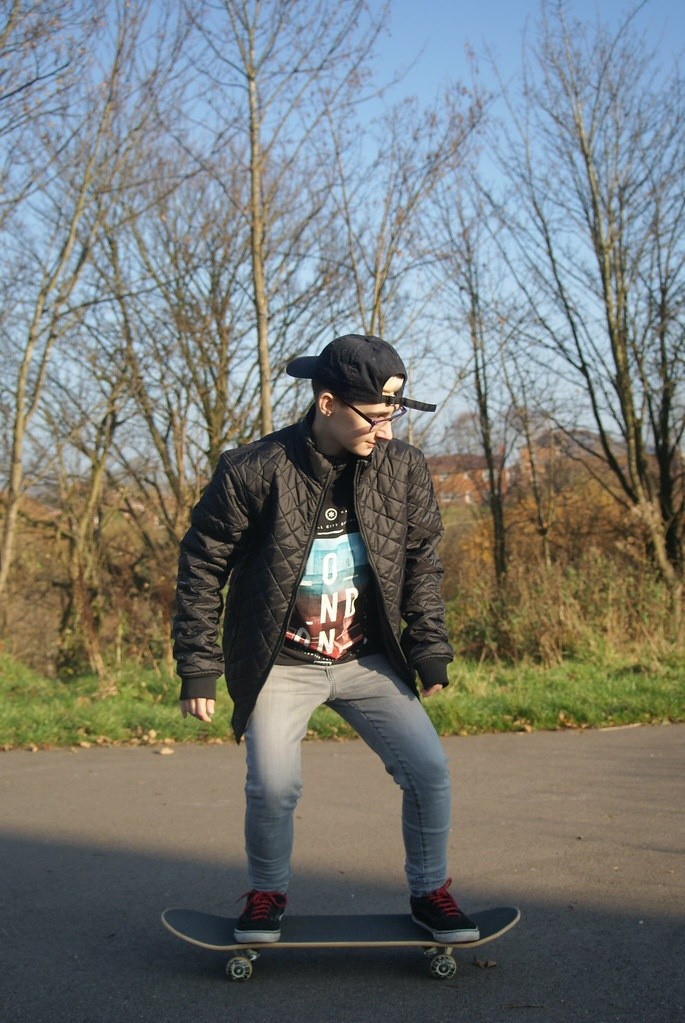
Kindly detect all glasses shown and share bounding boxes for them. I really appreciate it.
[330,392,407,432]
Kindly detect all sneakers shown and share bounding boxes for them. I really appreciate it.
[411,878,481,943]
[233,890,287,944]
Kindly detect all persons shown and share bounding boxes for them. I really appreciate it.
[173,330,481,942]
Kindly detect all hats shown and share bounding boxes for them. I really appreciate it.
[285,334,437,412]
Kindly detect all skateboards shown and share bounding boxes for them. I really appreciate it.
[161,906,521,982]
